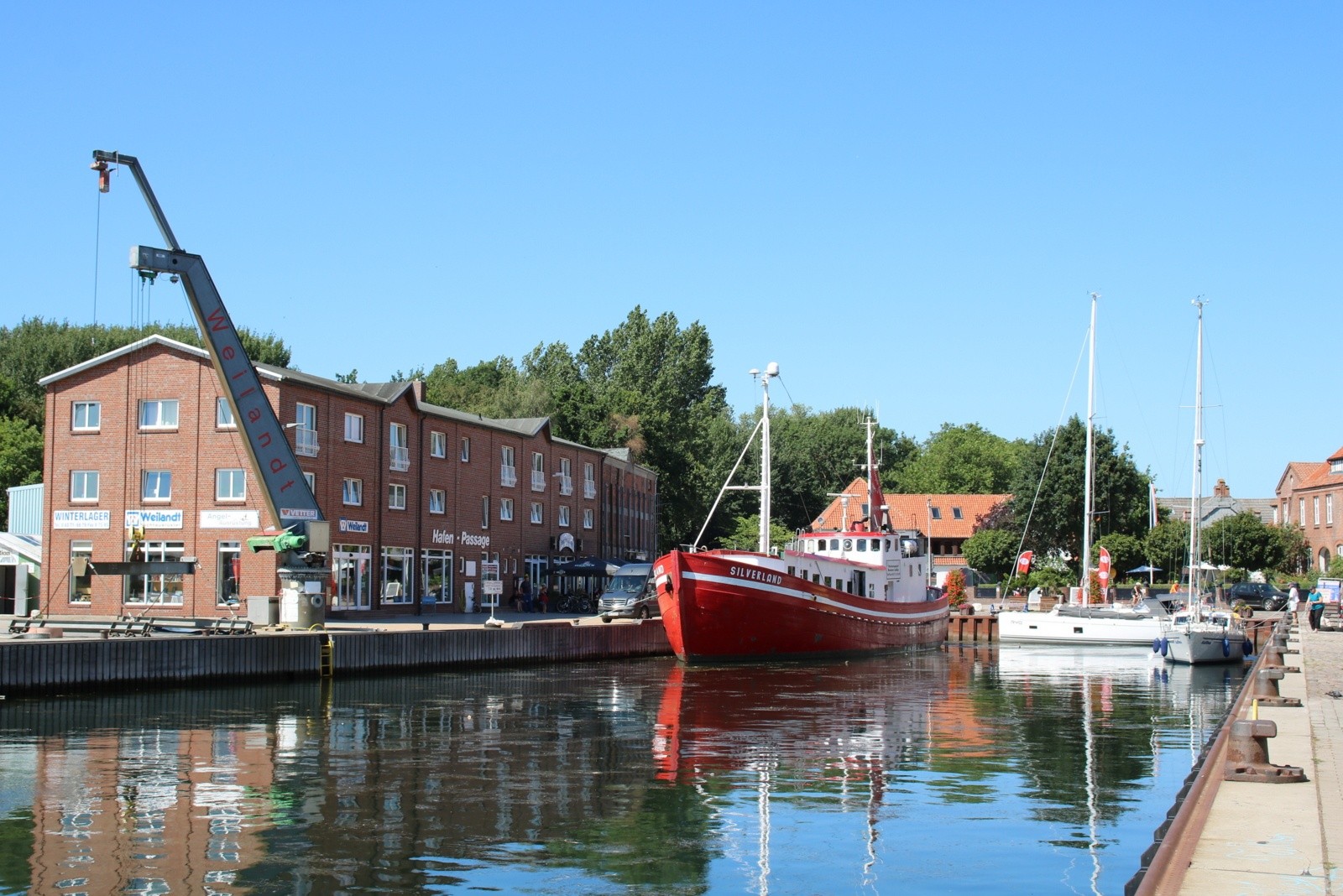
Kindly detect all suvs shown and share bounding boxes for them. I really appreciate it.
[1225,583,1289,612]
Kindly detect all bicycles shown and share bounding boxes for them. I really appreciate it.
[557,590,596,614]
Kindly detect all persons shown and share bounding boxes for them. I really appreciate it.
[1286,582,1300,624]
[1170,580,1183,609]
[638,579,651,598]
[1305,585,1324,632]
[1131,582,1142,607]
[623,578,632,591]
[508,590,523,613]
[538,587,548,614]
[520,576,536,613]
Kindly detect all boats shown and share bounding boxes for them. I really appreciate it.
[651,363,952,665]
[650,641,949,896]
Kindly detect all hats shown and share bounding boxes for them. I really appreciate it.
[1136,582,1142,585]
[1175,580,1179,583]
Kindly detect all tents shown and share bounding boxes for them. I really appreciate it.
[546,557,653,600]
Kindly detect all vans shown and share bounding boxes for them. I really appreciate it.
[598,563,663,624]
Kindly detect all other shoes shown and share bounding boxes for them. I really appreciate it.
[1311,628,1319,633]
[1294,621,1298,624]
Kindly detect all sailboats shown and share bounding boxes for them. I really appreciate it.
[988,290,1174,646]
[1147,293,1257,664]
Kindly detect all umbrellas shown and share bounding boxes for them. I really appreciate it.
[1125,565,1163,573]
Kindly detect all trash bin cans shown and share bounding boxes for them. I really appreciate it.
[246,595,280,627]
